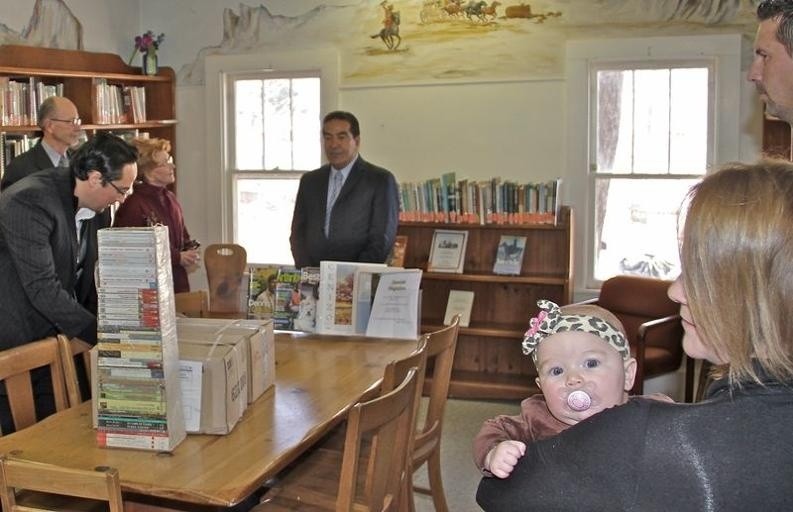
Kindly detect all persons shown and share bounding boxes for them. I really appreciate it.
[0,96,83,193]
[475,301,674,484]
[288,112,396,269]
[742,1,792,128]
[472,154,792,512]
[114,137,198,292]
[0,128,138,435]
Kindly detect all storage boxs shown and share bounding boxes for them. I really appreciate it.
[173,314,276,406]
[171,331,249,439]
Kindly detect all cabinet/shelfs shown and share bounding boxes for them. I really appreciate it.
[385,204,583,405]
[0,40,178,227]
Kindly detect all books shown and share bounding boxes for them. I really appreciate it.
[1,75,150,178]
[394,172,559,227]
[386,236,408,268]
[492,234,527,277]
[93,223,188,452]
[442,289,475,329]
[245,259,420,343]
[425,229,469,273]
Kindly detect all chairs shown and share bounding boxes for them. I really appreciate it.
[0,460,126,512]
[0,333,110,511]
[405,314,470,511]
[169,290,210,323]
[580,269,690,398]
[314,335,433,512]
[247,366,421,512]
[54,329,96,408]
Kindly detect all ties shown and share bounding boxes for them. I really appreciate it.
[325,172,342,238]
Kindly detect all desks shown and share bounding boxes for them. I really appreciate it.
[0,328,417,512]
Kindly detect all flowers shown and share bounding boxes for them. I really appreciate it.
[125,27,168,69]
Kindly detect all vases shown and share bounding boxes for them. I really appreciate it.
[142,52,160,76]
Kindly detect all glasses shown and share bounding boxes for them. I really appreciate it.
[50,118,81,126]
[85,168,129,194]
